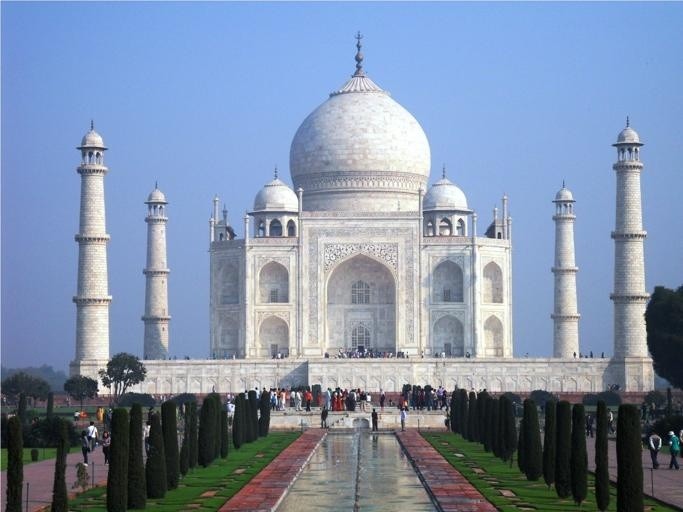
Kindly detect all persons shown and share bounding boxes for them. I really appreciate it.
[226,385,385,413]
[539,390,560,412]
[73,406,111,467]
[640,401,658,425]
[511,393,527,417]
[465,351,470,358]
[471,387,486,400]
[334,344,409,358]
[421,351,424,359]
[573,350,593,358]
[641,429,683,470]
[608,407,616,435]
[273,351,289,360]
[400,407,406,432]
[434,351,446,358]
[320,405,328,429]
[135,351,239,360]
[148,407,154,424]
[152,393,175,404]
[523,352,529,358]
[178,401,185,421]
[586,415,594,438]
[142,421,152,457]
[398,383,449,412]
[372,409,378,432]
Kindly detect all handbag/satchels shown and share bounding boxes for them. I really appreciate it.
[87,436,91,442]
